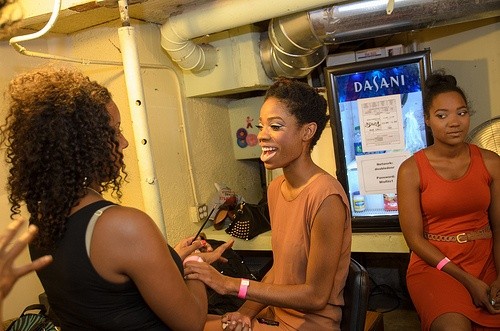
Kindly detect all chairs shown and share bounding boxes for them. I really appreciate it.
[339,257,370,331]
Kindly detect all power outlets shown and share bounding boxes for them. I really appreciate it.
[190,204,209,223]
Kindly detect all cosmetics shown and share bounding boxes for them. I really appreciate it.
[214,208,229,229]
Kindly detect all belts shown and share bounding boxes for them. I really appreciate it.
[423,225,492,243]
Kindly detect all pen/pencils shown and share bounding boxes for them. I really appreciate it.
[189,203,219,246]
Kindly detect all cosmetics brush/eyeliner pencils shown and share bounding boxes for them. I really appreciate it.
[191,204,217,245]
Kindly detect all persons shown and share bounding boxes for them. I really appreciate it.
[0,70,235,331]
[183,79,352,331]
[396,70,500,331]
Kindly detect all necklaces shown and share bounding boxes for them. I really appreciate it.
[83,186,103,197]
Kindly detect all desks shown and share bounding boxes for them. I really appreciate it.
[200,224,412,254]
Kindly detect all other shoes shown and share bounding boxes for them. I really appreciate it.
[213,196,236,230]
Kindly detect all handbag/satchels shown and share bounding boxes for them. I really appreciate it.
[224,196,271,240]
[5,304,61,331]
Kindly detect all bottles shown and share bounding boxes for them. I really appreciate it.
[353,190,365,212]
[383,193,399,211]
[354,126,365,160]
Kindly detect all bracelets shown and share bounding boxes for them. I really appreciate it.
[238,278,250,300]
[437,257,450,270]
[182,256,204,265]
